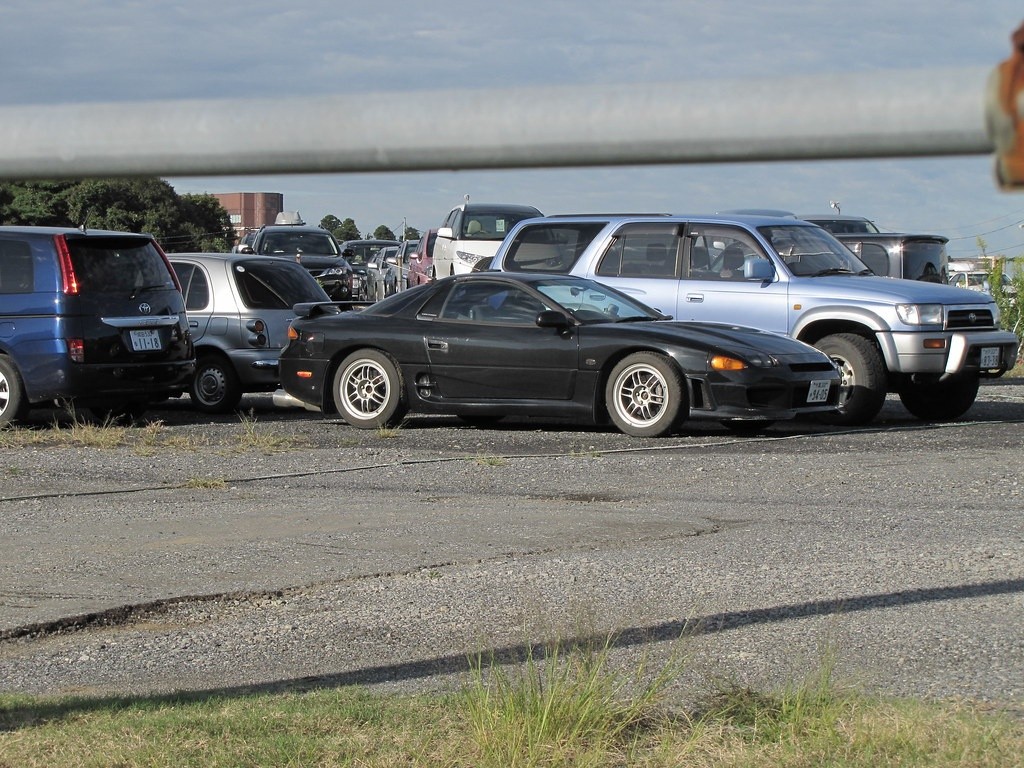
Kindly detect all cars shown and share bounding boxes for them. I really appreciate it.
[407,227,438,285]
[337,239,402,304]
[951,271,1015,308]
[434,204,566,280]
[137,252,338,417]
[230,210,355,311]
[388,241,420,296]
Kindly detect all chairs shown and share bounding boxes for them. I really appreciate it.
[465,219,481,236]
[469,304,497,321]
[691,246,746,279]
[603,242,673,275]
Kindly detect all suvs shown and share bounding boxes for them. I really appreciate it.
[473,212,1016,427]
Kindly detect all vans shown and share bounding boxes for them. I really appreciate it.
[698,210,950,284]
[0,222,198,434]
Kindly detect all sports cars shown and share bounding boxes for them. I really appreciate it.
[277,269,844,438]
